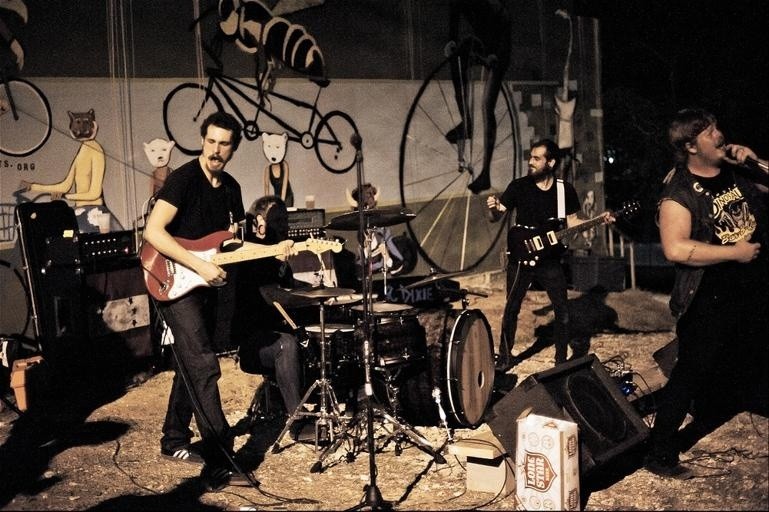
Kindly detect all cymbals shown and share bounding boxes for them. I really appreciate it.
[324,208,417,229]
[291,287,355,296]
[314,292,379,306]
[406,266,474,291]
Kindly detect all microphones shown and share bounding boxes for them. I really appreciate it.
[724,147,769,173]
[350,133,364,162]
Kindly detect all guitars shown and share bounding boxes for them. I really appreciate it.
[507,199,641,268]
[141,230,344,301]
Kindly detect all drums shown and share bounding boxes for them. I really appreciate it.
[352,303,416,316]
[351,318,411,367]
[368,309,495,427]
[302,323,357,370]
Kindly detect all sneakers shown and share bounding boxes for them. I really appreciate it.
[158,443,205,464]
[643,456,695,480]
[199,466,258,487]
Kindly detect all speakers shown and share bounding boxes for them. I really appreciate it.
[87,267,157,369]
[492,354,656,477]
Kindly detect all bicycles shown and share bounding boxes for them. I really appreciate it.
[161,13,361,174]
[0,188,66,340]
[0,74,53,158]
[398,0,541,274]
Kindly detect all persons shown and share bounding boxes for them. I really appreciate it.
[239,196,323,442]
[646,108,769,479]
[139,112,299,487]
[487,138,616,372]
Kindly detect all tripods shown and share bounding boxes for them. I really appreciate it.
[271,299,356,461]
[308,162,447,512]
[309,231,446,472]
